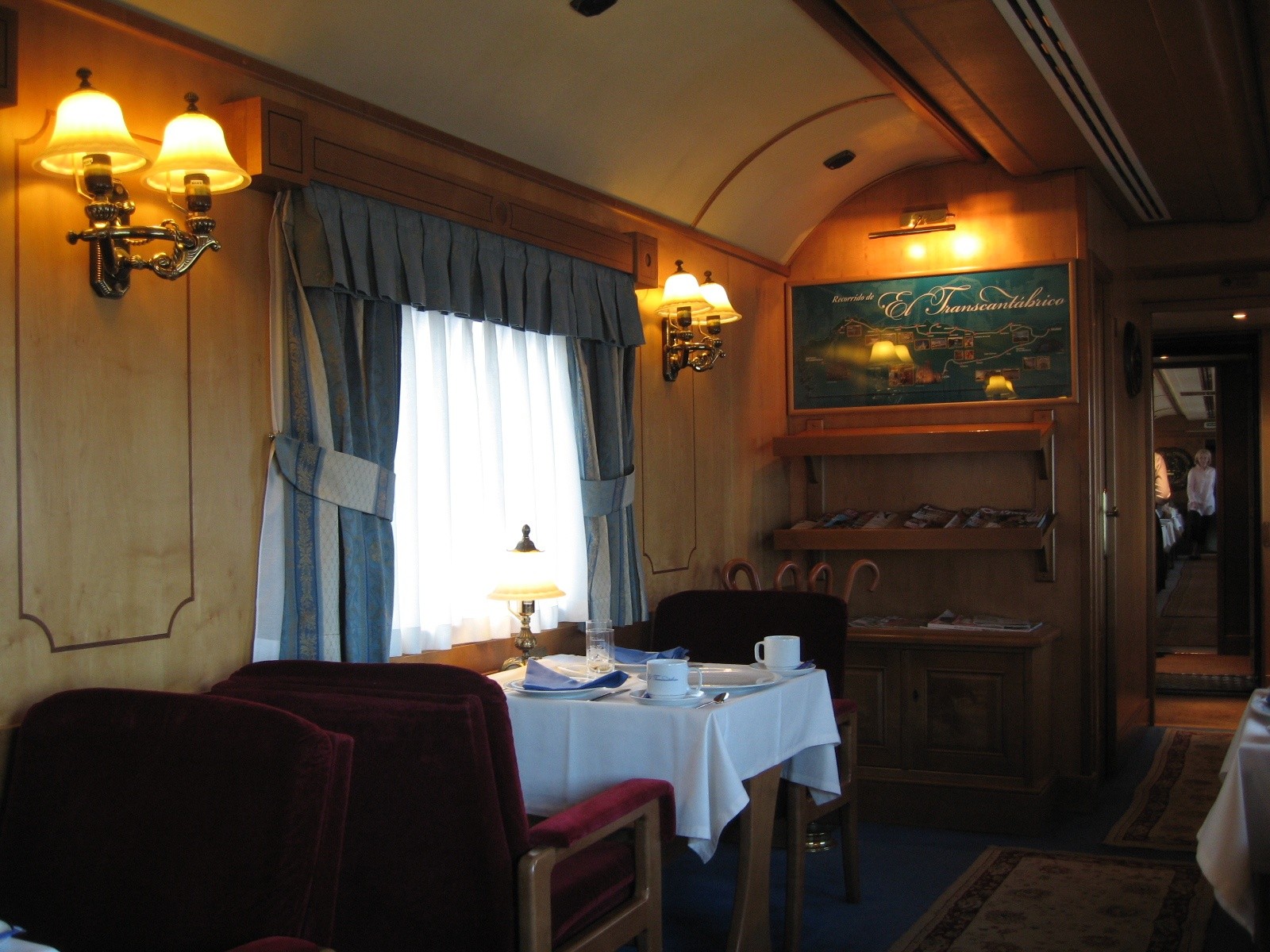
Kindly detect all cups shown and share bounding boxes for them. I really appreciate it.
[647,658,703,699]
[754,635,801,669]
[584,618,616,679]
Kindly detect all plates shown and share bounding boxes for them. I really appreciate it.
[503,678,609,697]
[629,688,705,706]
[637,663,782,693]
[750,661,818,675]
[1249,696,1270,718]
[616,656,690,673]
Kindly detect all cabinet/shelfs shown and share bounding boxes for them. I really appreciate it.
[774,410,1085,805]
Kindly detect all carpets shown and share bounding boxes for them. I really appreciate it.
[882,848,1226,952]
[1108,726,1255,869]
[1154,552,1219,647]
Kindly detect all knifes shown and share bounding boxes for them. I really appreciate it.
[588,688,630,702]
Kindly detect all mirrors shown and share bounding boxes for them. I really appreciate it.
[1150,356,1254,681]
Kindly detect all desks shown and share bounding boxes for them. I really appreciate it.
[482,652,844,952]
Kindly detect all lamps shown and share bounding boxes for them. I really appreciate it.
[869,203,957,240]
[26,66,257,296]
[649,262,749,378]
[489,521,567,670]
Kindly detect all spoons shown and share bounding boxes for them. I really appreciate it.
[693,692,733,709]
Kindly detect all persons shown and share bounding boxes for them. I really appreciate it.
[1185,448,1215,554]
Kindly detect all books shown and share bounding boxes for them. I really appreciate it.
[849,606,1044,635]
[791,501,1049,529]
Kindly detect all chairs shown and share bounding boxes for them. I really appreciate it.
[0,688,343,952]
[641,589,856,893]
[198,659,676,952]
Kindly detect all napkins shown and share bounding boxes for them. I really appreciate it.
[520,640,689,689]
